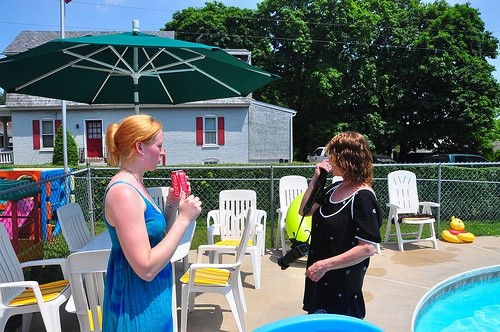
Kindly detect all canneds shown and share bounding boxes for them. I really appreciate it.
[171,170,189,198]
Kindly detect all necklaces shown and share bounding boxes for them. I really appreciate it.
[123,167,150,195]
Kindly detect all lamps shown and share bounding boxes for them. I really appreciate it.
[76,123,79,128]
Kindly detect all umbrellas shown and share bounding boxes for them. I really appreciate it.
[0,21,281,115]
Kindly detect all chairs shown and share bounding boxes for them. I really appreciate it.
[145,174,344,332]
[384,171,441,252]
[0,202,111,331]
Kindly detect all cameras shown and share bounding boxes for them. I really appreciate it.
[277,238,310,270]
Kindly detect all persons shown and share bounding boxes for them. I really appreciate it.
[102,113,202,332]
[298,132,383,319]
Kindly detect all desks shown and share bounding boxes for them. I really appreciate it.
[77,220,196,311]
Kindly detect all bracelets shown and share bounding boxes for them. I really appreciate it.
[166,202,179,209]
